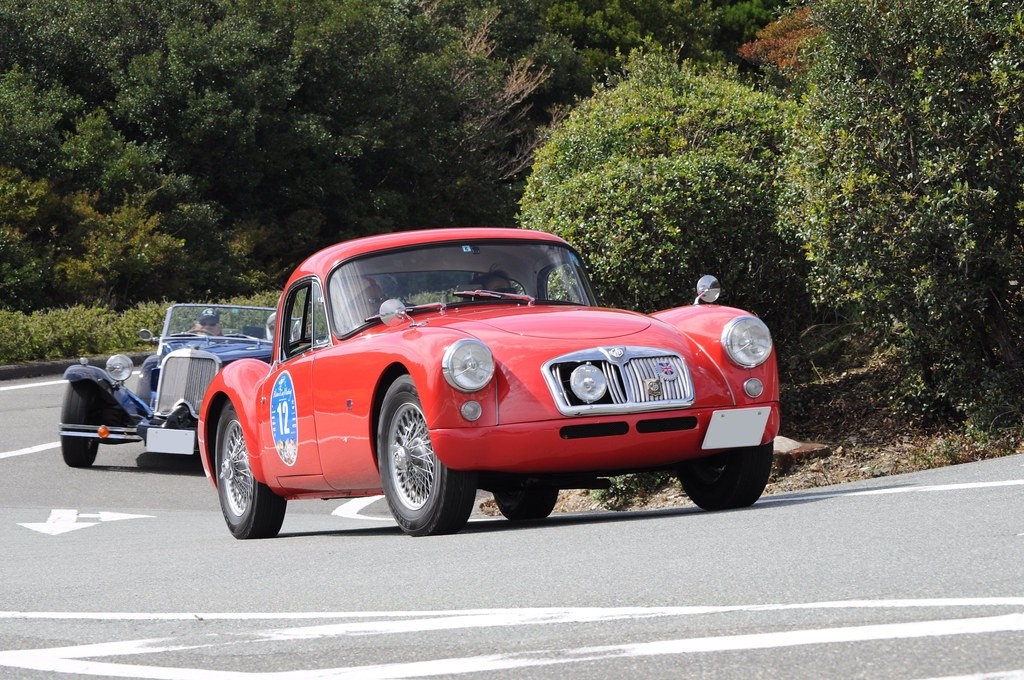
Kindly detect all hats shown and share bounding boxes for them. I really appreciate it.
[197,307,219,322]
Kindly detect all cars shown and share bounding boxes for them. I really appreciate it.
[198,225,780,540]
[58,304,328,469]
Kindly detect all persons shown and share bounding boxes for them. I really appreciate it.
[461,270,516,302]
[336,276,383,329]
[266,312,277,341]
[194,308,223,336]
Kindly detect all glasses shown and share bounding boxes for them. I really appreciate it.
[494,288,517,295]
[269,325,274,329]
[198,321,220,326]
[358,295,388,304]
